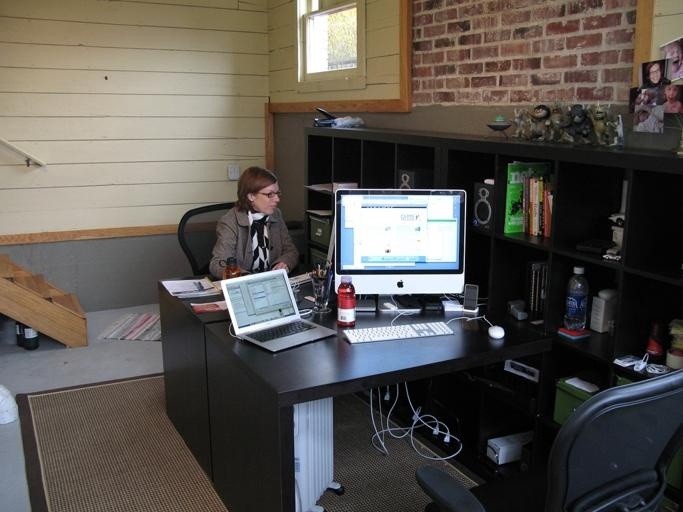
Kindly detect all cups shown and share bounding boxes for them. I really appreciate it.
[309,273,332,313]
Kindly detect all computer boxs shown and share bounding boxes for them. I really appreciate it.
[456,363,518,466]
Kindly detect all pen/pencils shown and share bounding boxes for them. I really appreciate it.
[198,282,204,290]
[193,281,200,290]
[312,262,333,277]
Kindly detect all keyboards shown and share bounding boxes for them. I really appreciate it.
[342,321,455,344]
[288,269,317,287]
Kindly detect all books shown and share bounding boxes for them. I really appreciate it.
[502,160,552,241]
[305,179,357,218]
[521,257,546,313]
[160,277,221,300]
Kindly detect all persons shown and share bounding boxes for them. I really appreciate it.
[630,89,642,112]
[207,165,300,280]
[643,62,670,88]
[663,43,682,81]
[662,86,683,113]
[642,89,662,113]
[634,110,663,133]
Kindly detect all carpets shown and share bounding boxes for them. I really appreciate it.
[17,370,483,512]
[100,312,161,341]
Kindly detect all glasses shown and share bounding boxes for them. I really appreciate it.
[259,192,281,198]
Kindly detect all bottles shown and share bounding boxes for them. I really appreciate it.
[15,322,39,349]
[664,344,682,373]
[222,257,240,279]
[565,266,588,330]
[645,325,662,362]
[336,275,356,327]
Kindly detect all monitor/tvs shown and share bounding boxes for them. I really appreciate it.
[324,208,376,311]
[334,189,467,313]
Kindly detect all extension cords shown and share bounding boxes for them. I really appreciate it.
[377,389,454,449]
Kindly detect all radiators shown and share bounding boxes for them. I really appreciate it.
[294,397,345,512]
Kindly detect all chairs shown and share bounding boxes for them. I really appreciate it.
[177,204,238,277]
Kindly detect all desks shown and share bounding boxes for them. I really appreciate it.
[205,310,550,512]
[157,273,336,512]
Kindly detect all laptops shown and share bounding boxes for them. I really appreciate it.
[220,268,339,353]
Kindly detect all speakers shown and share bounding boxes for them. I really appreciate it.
[398,169,434,189]
[472,175,507,234]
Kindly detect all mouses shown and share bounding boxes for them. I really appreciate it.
[488,326,505,340]
